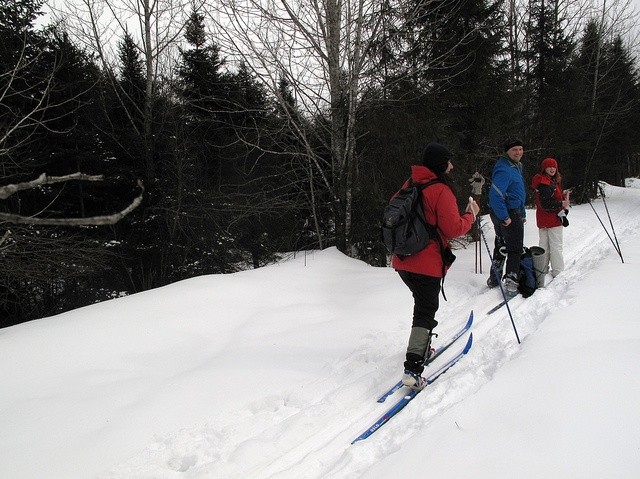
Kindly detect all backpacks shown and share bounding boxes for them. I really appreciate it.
[520,248,534,297]
[381,177,446,261]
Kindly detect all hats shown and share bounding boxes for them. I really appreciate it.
[423,142,454,173]
[503,139,523,152]
[541,158,558,176]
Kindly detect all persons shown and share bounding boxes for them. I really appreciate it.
[531,158,571,277]
[486,136,527,297]
[381,142,480,391]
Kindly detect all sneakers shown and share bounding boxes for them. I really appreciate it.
[402,364,427,389]
[505,283,516,297]
[486,277,500,289]
[422,348,435,366]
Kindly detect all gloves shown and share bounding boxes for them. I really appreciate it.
[565,209,569,215]
[558,210,569,227]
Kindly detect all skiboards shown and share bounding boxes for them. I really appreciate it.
[351,309,473,446]
[487,290,521,316]
